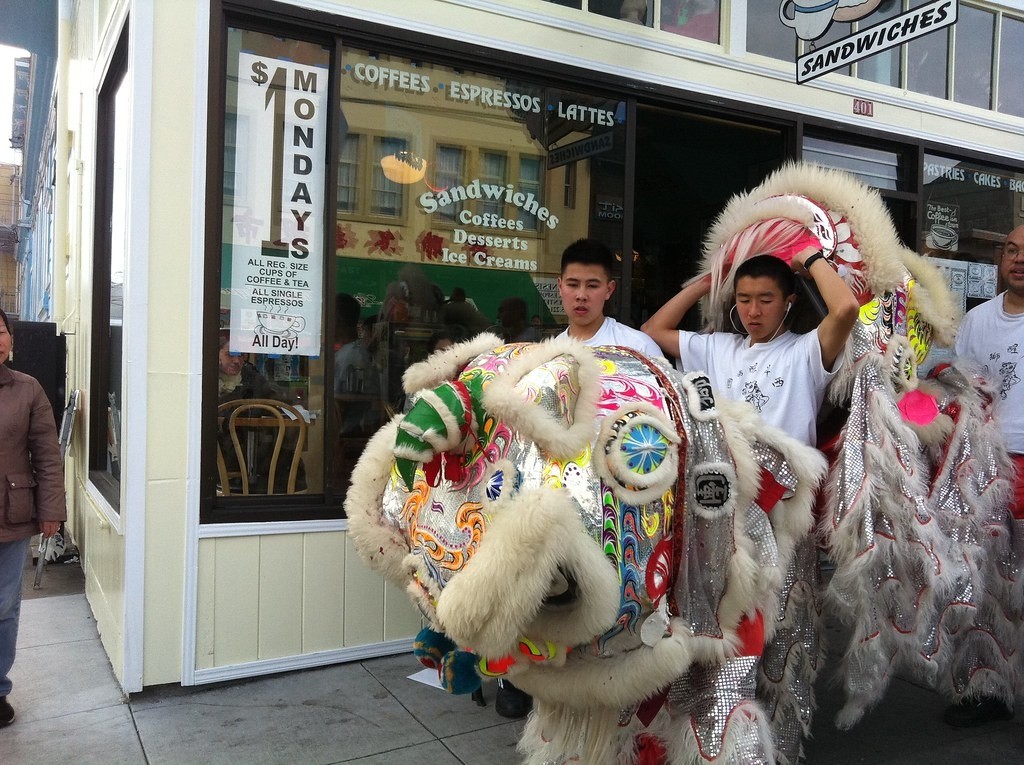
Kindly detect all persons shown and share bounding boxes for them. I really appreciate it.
[497,296,545,343]
[216,331,274,482]
[642,245,860,449]
[391,338,416,411]
[438,285,492,328]
[429,332,458,355]
[0,305,67,726]
[953,225,1024,563]
[550,239,666,362]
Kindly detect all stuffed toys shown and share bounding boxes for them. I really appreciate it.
[701,161,1024,728]
[342,329,828,764]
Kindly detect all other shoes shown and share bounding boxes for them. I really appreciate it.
[0,696,16,729]
[944,696,983,727]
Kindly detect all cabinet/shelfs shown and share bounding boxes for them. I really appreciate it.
[372,322,434,428]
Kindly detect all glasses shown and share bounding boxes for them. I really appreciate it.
[1000,247,1024,260]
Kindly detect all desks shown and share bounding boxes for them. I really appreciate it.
[218,416,307,494]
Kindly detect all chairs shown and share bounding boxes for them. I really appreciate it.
[216,398,306,495]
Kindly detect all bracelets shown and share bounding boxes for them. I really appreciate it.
[804,251,823,270]
